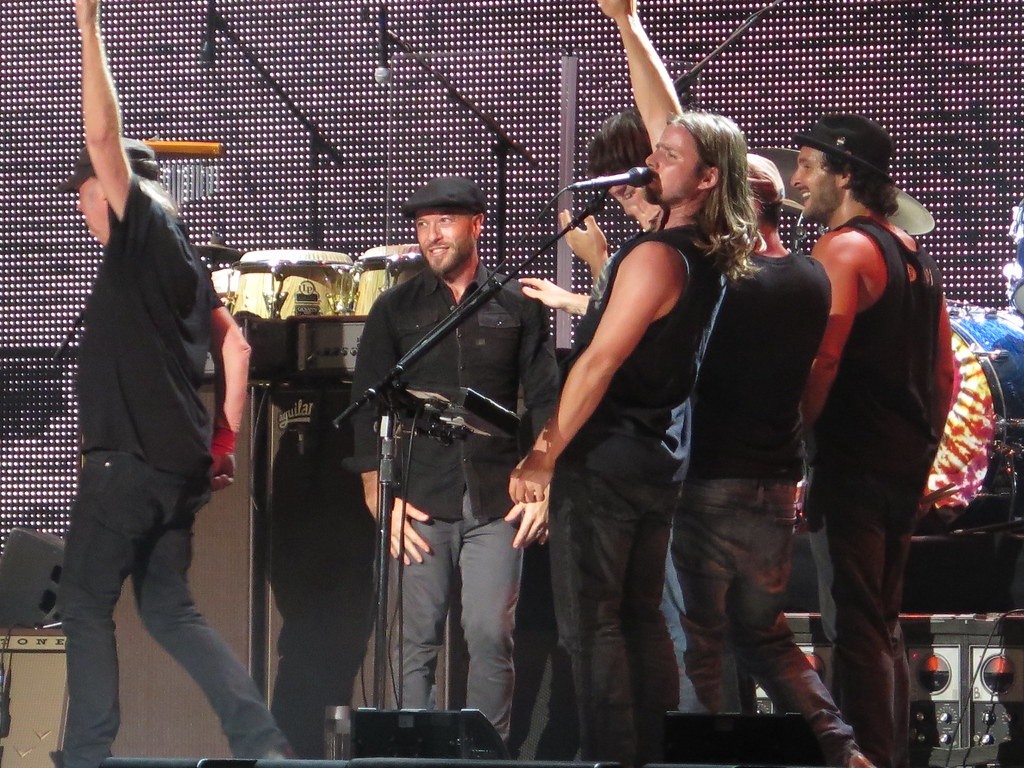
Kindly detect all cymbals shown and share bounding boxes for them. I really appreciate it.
[748,147,935,237]
[192,240,239,265]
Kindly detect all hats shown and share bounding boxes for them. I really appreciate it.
[56,139,158,194]
[795,112,897,187]
[403,175,485,216]
[745,152,785,205]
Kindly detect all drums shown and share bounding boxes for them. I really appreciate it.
[213,268,239,311]
[357,246,431,319]
[232,249,353,326]
[925,299,1024,529]
[1007,198,1023,314]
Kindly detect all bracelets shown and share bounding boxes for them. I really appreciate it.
[212,425,238,455]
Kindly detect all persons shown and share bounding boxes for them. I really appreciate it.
[790,114,955,768]
[353,177,561,751]
[51,0,289,768]
[520,107,878,768]
[509,0,759,761]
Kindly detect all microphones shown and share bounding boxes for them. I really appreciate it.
[568,167,653,191]
[0,662,6,716]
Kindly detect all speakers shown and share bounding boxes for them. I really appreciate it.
[0,628,71,768]
[266,381,403,761]
[659,711,825,767]
[0,527,64,629]
[101,383,258,760]
